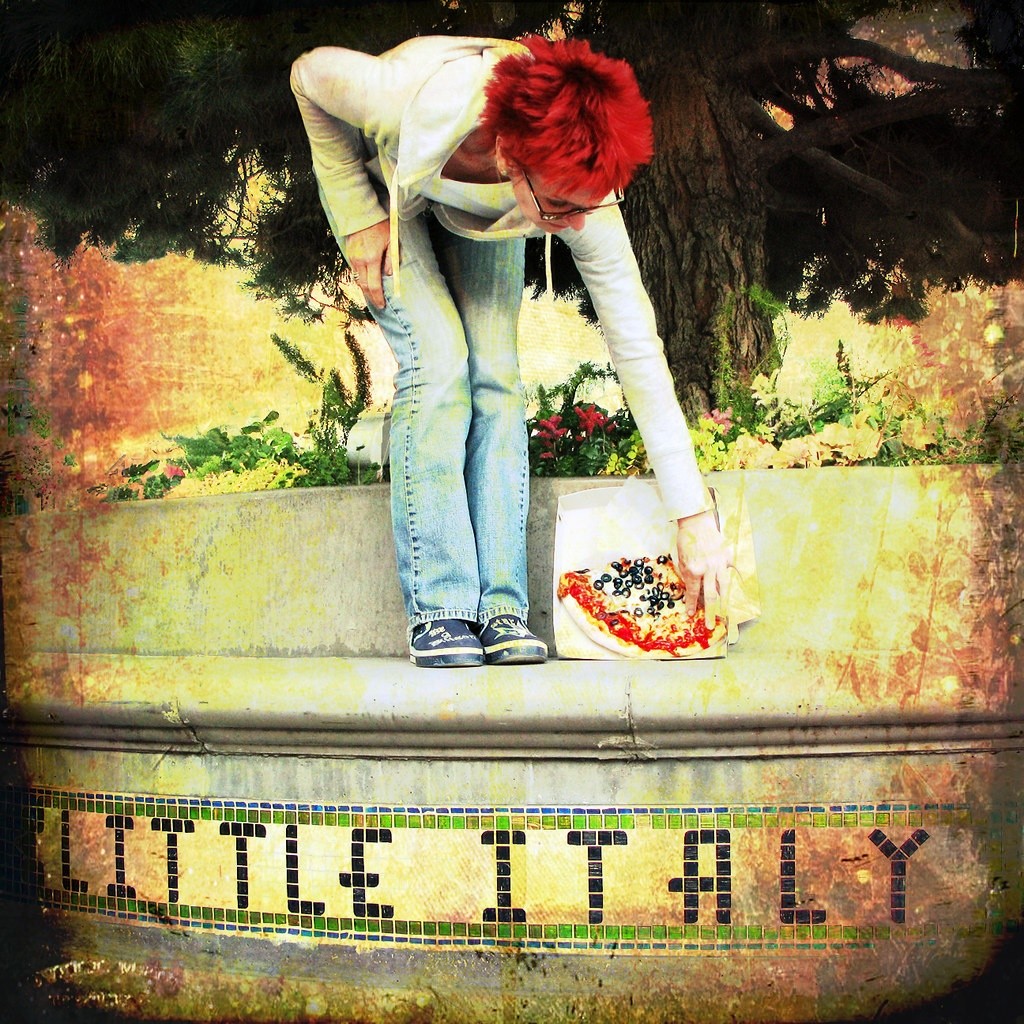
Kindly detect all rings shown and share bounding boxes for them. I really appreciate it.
[354,272,359,280]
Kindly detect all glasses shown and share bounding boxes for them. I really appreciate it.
[523,168,625,221]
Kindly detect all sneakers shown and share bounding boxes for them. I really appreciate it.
[409,620,483,668]
[477,614,549,664]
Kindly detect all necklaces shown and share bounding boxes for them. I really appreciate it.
[495,155,503,183]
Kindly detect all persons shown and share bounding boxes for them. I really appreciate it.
[290,35,735,668]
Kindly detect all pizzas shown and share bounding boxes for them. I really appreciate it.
[557,552,727,661]
